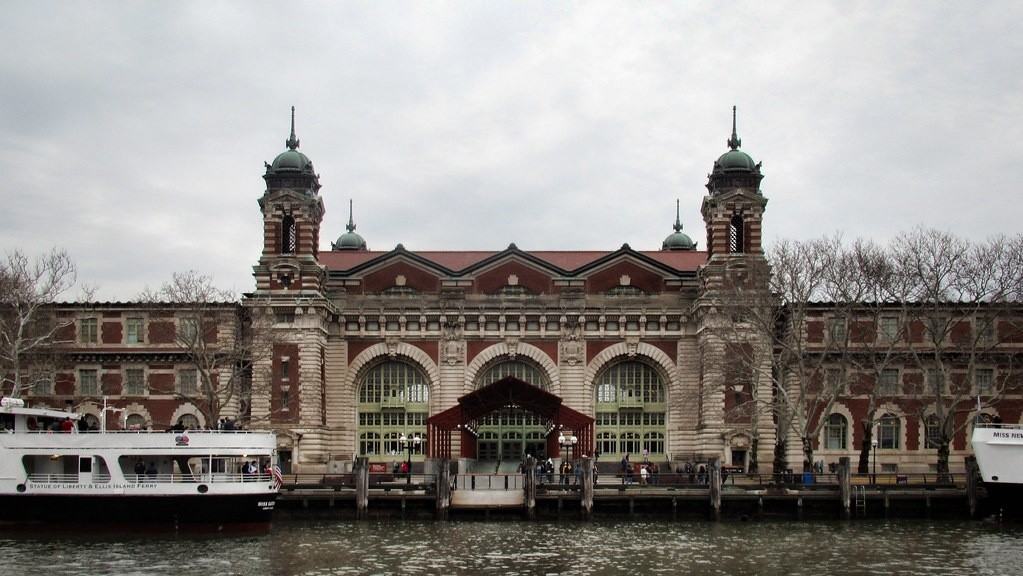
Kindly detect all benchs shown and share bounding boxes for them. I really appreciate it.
[816,475,840,485]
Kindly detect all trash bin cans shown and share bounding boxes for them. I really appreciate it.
[802,472,813,483]
[781,469,793,483]
[794,474,802,484]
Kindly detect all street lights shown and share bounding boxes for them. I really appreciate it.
[558,432,578,492]
[398,433,421,484]
[871,440,878,484]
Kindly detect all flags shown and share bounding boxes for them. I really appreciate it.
[978,396,982,413]
[274,458,283,490]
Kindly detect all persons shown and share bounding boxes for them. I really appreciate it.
[134,458,146,483]
[237,459,272,482]
[813,460,835,474]
[991,414,1002,428]
[400,461,408,477]
[393,461,399,478]
[643,448,648,461]
[146,462,159,483]
[559,461,580,484]
[621,454,634,484]
[593,465,598,484]
[166,416,252,433]
[980,415,990,427]
[593,448,601,462]
[640,461,658,485]
[685,461,728,484]
[42,416,148,433]
[517,454,554,482]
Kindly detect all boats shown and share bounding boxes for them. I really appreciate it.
[971,396,1023,515]
[0,397,282,521]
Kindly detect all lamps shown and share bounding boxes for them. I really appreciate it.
[77,355,82,362]
[100,355,104,362]
[130,355,137,362]
[122,355,126,362]
[168,355,173,361]
[176,355,181,361]
[160,356,165,363]
[115,355,120,362]
[184,355,189,362]
[145,356,150,362]
[84,356,90,361]
[92,355,97,361]
[69,356,76,361]
[138,357,142,362]
[153,355,159,362]
[192,354,196,362]
[108,356,112,362]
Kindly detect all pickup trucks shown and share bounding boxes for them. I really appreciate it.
[321,462,395,490]
